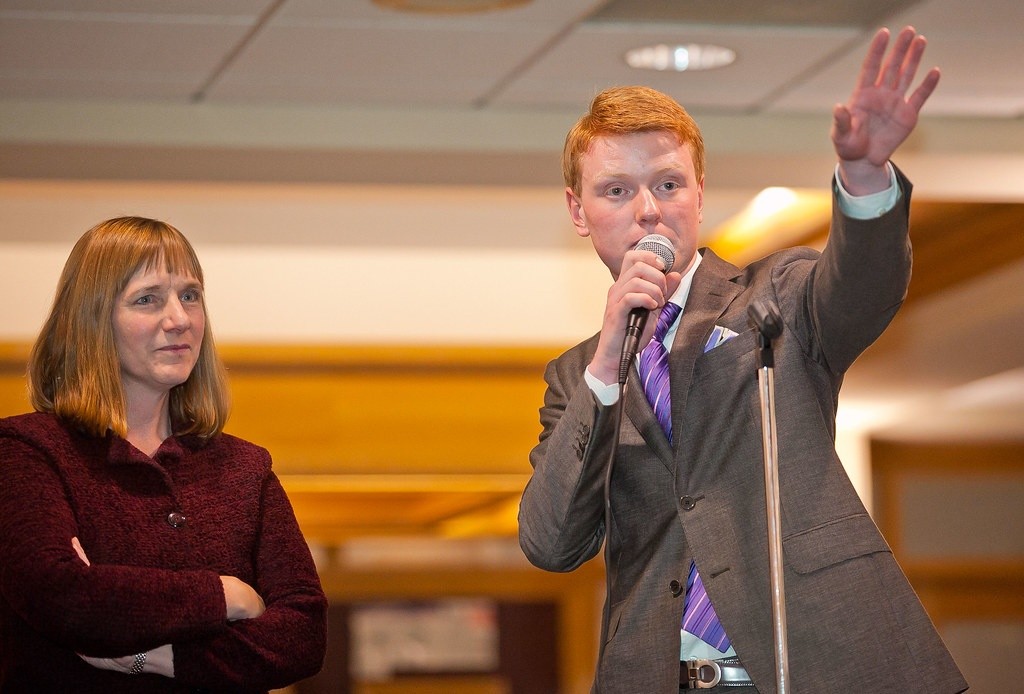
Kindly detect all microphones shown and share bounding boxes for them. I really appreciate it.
[618,233,677,384]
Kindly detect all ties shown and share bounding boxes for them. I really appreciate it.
[638,300,731,654]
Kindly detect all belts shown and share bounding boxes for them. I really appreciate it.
[679,656,754,690]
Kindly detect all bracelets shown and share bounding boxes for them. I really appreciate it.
[129,653,148,675]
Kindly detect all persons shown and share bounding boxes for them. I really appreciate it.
[0,213,334,694]
[517,23,973,694]
